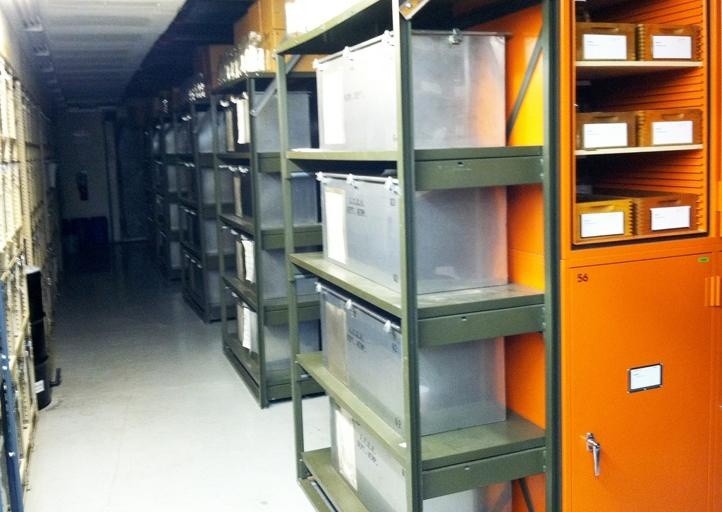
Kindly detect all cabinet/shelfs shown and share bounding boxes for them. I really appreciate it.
[172,100,238,324]
[211,72,326,410]
[274,0,556,512]
[0,55,61,512]
[562,0,722,512]
[149,113,183,282]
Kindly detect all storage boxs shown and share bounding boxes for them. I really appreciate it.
[247,3,264,31]
[576,194,633,240]
[262,28,318,72]
[203,43,231,84]
[233,15,249,45]
[576,22,635,61]
[638,110,703,146]
[260,0,286,30]
[634,191,697,235]
[576,112,638,149]
[637,24,702,59]
